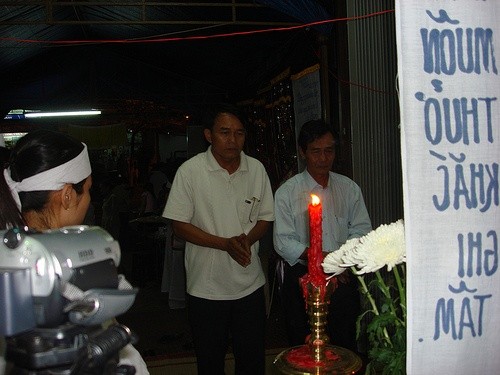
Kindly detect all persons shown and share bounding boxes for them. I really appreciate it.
[272,118,374,348]
[0,129,153,375]
[100,170,186,311]
[162,106,276,375]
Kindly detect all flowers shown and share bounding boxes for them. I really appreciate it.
[321,218,407,375]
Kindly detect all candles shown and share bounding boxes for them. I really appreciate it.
[308,203,323,280]
